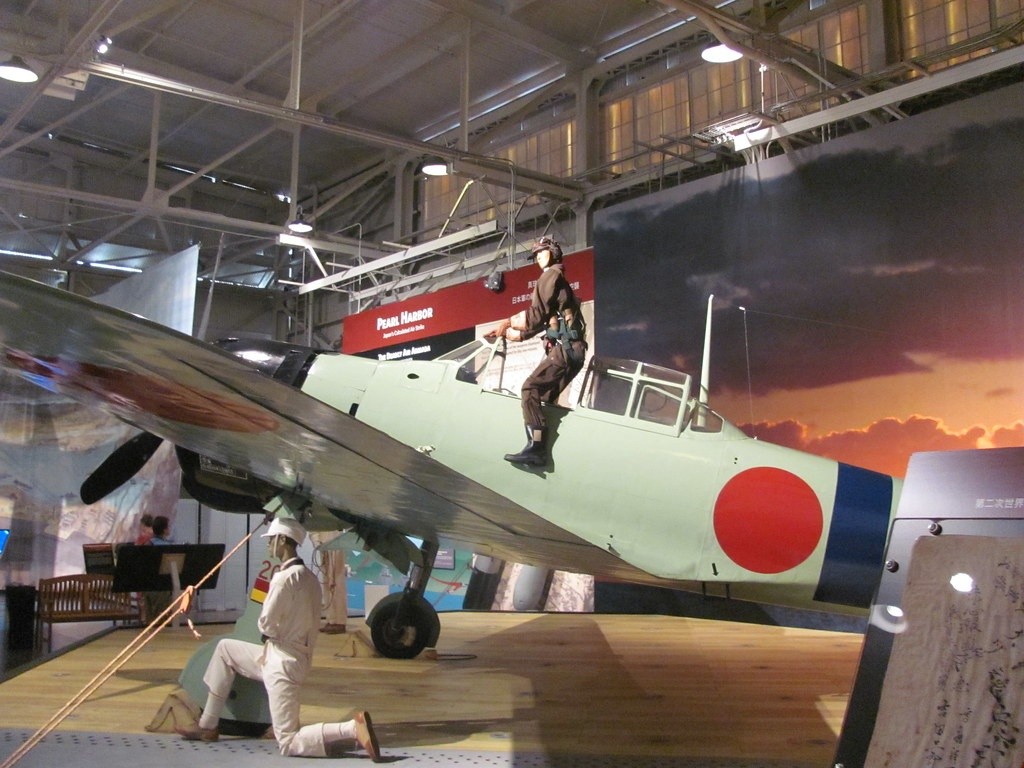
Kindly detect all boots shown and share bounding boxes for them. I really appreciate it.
[503,423,549,466]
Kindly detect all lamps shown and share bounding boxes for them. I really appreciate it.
[0,56,39,83]
[421,157,448,176]
[288,213,313,232]
[701,41,743,63]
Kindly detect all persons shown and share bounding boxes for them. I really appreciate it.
[484,237,588,466]
[177,516,384,763]
[136,514,175,626]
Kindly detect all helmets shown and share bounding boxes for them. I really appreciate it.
[532,237,563,262]
[260,517,308,548]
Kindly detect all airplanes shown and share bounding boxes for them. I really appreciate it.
[1,268,908,737]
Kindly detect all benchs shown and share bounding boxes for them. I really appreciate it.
[34,573,141,654]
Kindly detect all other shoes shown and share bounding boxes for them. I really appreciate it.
[172,723,218,743]
[354,710,382,762]
